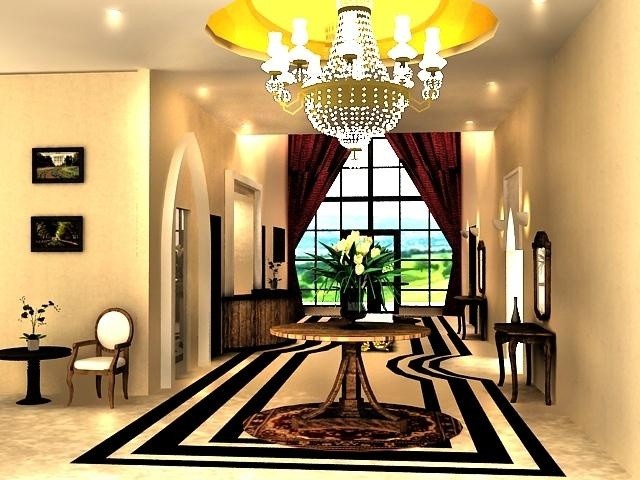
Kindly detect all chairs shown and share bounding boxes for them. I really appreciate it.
[66,308,134,408]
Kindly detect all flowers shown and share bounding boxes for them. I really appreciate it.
[18,295,61,340]
[303,230,415,310]
[268,262,282,283]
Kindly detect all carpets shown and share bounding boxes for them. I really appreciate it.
[241,401,463,453]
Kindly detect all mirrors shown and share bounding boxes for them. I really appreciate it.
[532,231,551,320]
[477,240,485,295]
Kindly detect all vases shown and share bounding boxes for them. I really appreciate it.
[339,287,369,330]
[271,281,278,290]
[26,340,40,351]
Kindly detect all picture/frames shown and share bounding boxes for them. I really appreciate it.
[32,147,84,183]
[31,216,83,252]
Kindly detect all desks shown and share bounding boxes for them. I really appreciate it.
[270,322,431,432]
[453,296,487,340]
[0,346,71,405]
[494,323,555,406]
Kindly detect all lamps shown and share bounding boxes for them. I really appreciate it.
[260,0,447,171]
